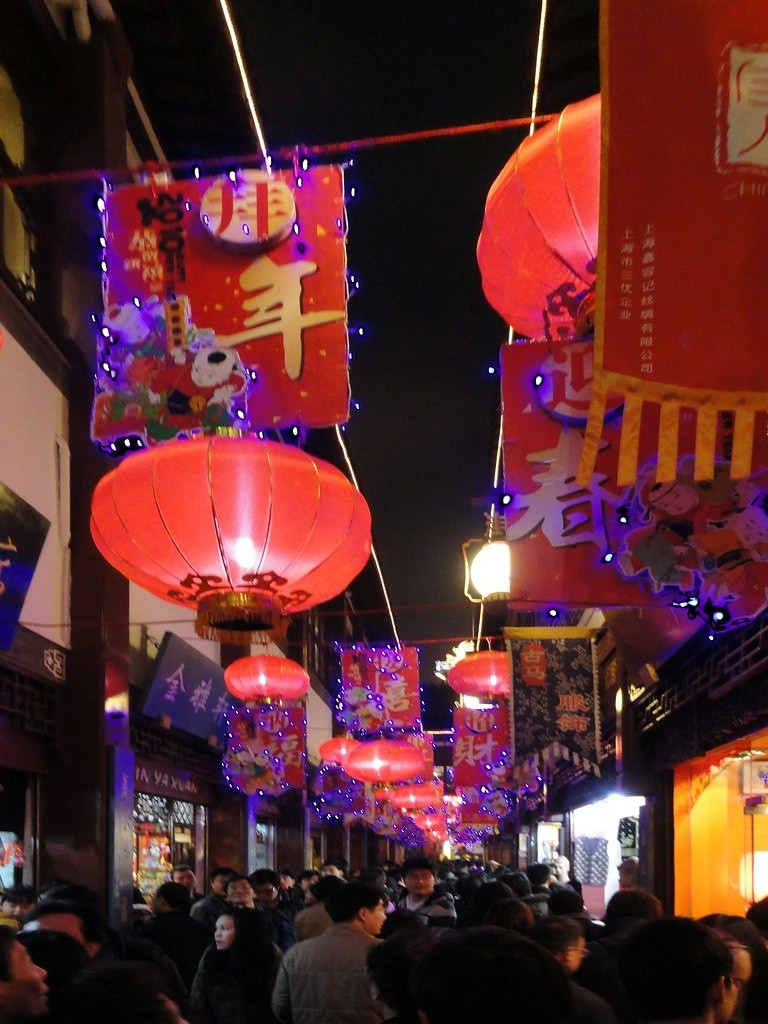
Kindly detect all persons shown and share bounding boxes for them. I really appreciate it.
[0,856,768,1024]
[553,854,582,896]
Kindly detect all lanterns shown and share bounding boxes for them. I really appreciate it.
[224,654,310,704]
[342,739,425,784]
[447,650,511,702]
[374,780,449,843]
[90,428,373,644]
[476,90,601,342]
[319,736,360,762]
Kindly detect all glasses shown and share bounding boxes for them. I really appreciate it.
[255,886,275,895]
[569,947,590,958]
[727,943,748,954]
[715,976,746,993]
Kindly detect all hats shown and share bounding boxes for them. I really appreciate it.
[312,876,346,900]
[600,889,663,927]
